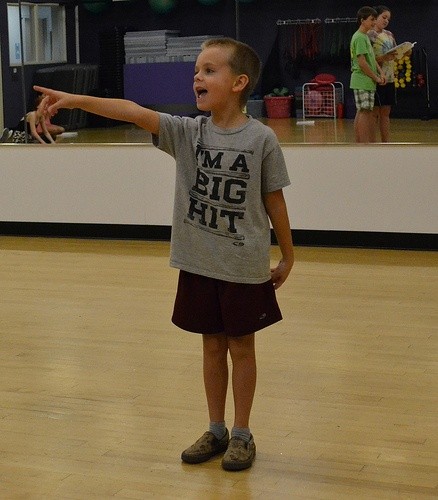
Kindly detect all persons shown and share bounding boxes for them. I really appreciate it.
[350,6,386,143]
[15,93,64,144]
[368,7,412,143]
[34,38,295,470]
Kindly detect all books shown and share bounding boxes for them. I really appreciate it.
[384,41,417,59]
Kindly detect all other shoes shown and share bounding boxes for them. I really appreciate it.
[181,427,230,463]
[223,435,257,470]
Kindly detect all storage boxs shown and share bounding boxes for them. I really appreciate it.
[302,82,344,120]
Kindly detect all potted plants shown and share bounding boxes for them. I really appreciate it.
[264,87,293,119]
